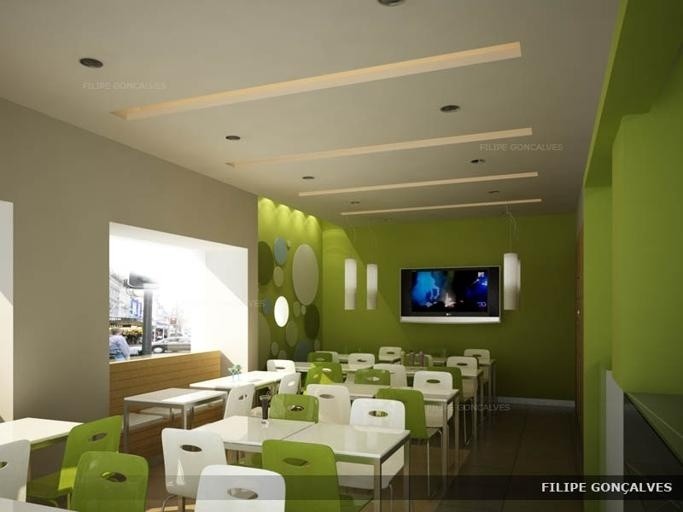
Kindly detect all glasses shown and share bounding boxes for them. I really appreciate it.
[344,215,357,310]
[366,216,378,310]
[504,206,521,310]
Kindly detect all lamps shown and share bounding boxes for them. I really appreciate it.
[504,206,521,310]
[366,216,378,310]
[344,215,357,310]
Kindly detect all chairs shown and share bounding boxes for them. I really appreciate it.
[0,346,491,512]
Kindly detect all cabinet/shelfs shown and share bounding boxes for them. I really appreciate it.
[399,349,405,369]
[407,351,414,369]
[418,350,423,368]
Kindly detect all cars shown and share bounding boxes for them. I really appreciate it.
[258,394,271,424]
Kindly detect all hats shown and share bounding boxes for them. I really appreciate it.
[150,336,190,353]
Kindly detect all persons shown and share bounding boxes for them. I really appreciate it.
[110,329,132,360]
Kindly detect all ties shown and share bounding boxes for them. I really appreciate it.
[400,265,501,324]
